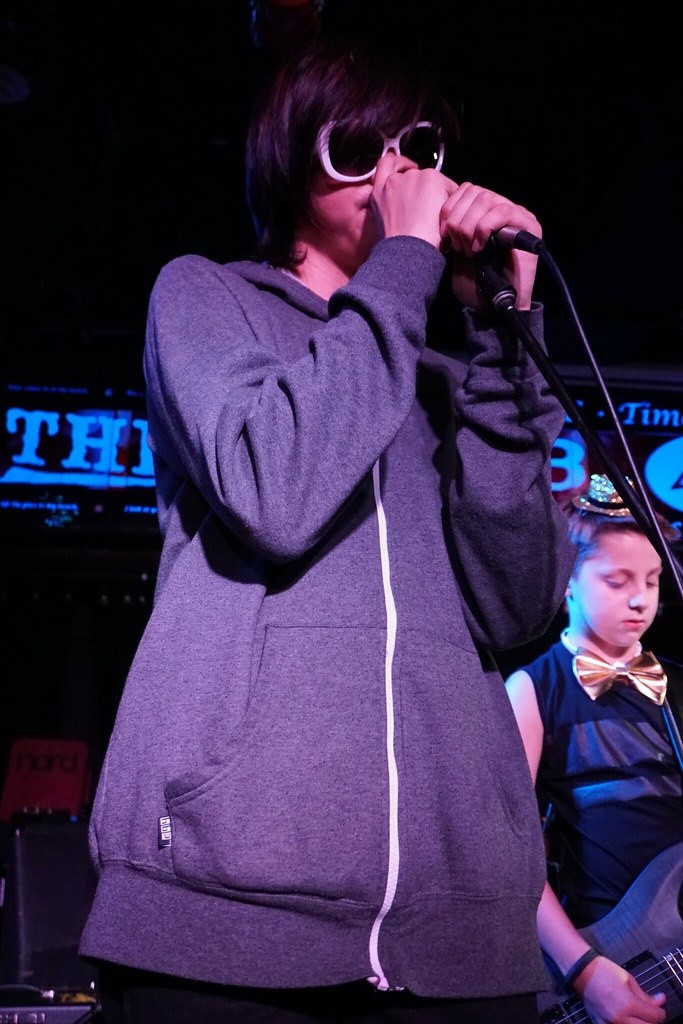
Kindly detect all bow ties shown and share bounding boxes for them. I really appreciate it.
[562,633,667,705]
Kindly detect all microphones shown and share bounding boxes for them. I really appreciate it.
[492,225,546,255]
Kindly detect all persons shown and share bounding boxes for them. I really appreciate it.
[505,473,683,1024]
[76,42,576,1024]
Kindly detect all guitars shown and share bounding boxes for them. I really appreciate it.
[531,839,683,1024]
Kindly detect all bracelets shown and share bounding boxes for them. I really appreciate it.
[564,948,603,986]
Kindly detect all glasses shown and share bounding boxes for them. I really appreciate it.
[310,117,444,185]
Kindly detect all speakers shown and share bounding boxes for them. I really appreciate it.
[0,827,107,1008]
